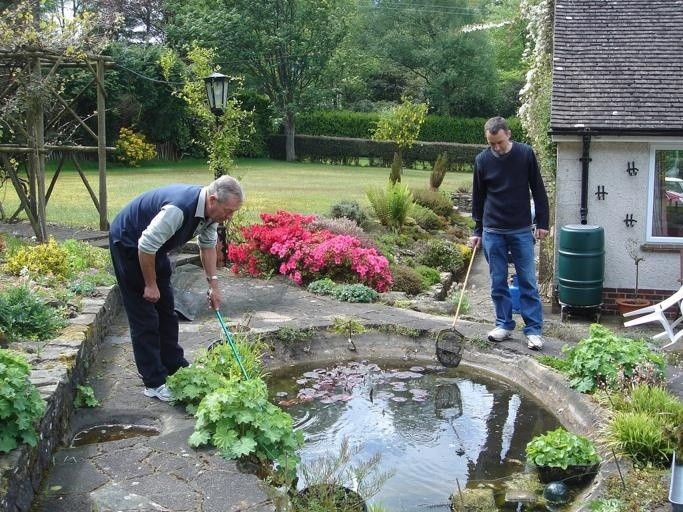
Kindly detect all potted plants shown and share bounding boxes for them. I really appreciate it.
[289,433,395,511]
[524,425,603,494]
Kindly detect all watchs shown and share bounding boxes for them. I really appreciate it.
[207,276,218,280]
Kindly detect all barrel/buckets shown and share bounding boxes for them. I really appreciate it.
[559,224,605,306]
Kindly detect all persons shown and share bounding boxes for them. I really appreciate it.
[108,173,244,404]
[469,117,549,352]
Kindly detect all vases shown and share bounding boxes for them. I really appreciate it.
[615,297,650,316]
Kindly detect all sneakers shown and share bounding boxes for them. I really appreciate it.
[526,334,544,351]
[487,327,514,342]
[143,385,179,402]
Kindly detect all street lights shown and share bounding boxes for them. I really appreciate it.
[198,63,234,266]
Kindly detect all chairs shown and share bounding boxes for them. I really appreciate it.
[622,284,683,349]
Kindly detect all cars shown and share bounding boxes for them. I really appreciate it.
[664,177,682,206]
